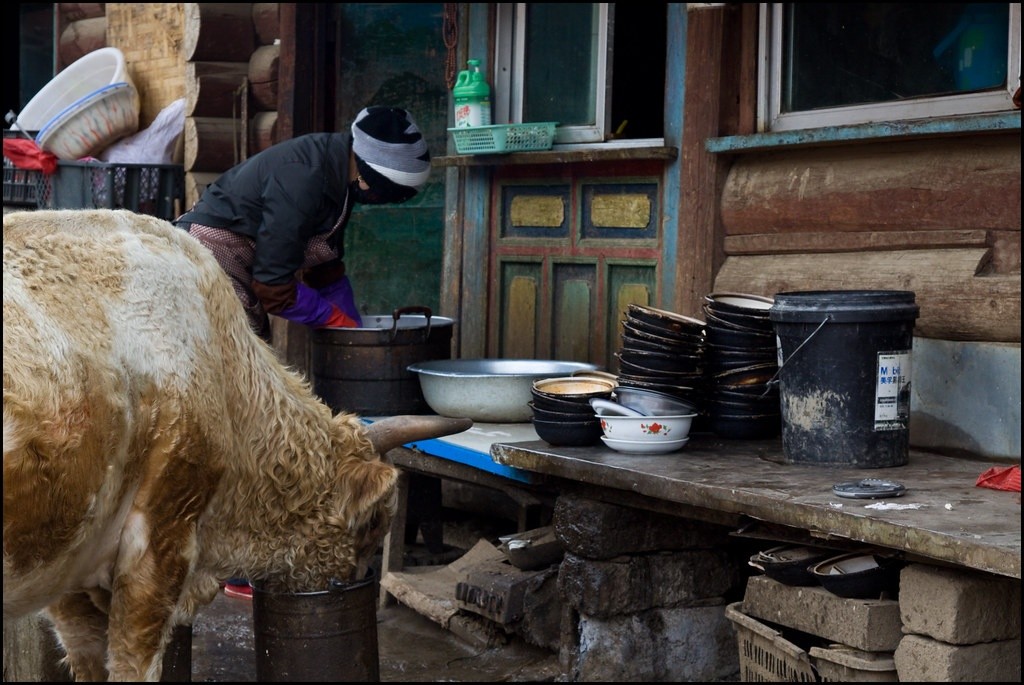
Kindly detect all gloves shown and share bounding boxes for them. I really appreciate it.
[278,281,358,328]
[316,278,364,328]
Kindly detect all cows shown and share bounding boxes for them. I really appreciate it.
[3,210,474,682]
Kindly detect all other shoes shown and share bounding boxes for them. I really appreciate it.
[224,583,253,600]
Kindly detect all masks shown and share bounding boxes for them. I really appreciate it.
[350,177,387,206]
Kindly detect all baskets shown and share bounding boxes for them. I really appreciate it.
[446,122,558,155]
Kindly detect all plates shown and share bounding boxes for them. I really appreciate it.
[600,435,690,454]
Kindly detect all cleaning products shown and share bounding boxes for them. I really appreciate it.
[452,59,492,128]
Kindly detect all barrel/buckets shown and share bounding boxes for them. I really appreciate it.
[766,290,921,469]
[250,570,380,683]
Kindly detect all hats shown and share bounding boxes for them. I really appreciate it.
[351,105,431,187]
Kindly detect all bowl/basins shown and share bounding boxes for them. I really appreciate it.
[406,359,598,423]
[529,291,778,446]
[8,45,140,160]
[748,545,889,599]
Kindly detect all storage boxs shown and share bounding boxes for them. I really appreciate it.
[3,129,182,222]
[726,603,901,682]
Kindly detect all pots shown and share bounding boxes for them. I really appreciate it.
[310,306,455,416]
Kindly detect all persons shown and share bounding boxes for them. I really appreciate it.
[170,107,432,598]
